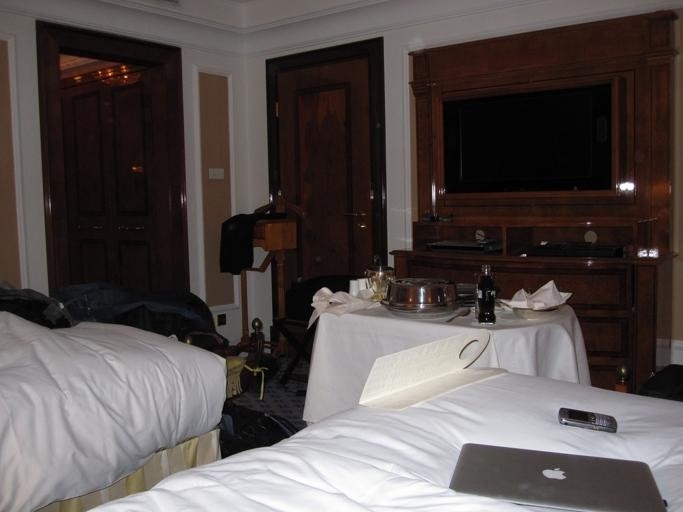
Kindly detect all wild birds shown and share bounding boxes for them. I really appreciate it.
[447,442,669,512]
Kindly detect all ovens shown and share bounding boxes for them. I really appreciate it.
[0,308,226,512]
[87,363,683,512]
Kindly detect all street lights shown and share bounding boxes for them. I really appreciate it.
[183,326,228,354]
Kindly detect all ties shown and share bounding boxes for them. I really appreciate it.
[442,80,614,197]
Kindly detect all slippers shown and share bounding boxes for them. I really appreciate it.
[219,399,297,458]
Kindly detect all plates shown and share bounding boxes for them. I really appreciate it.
[226,217,299,372]
[389,44,683,392]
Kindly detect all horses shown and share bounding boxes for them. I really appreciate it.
[557,406,618,433]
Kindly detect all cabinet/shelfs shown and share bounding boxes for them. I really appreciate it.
[616,359,631,385]
[477,264,497,325]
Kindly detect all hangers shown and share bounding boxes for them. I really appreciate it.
[445,309,470,322]
